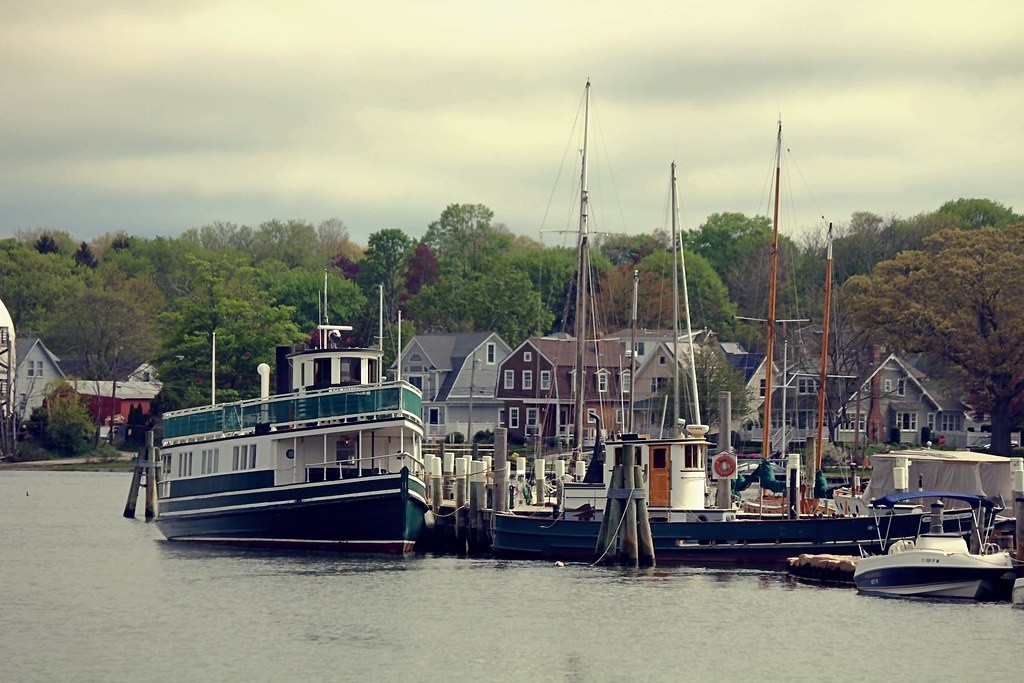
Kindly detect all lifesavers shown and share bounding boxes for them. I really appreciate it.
[715,456,735,476]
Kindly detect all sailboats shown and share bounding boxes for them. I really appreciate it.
[490,79,1003,566]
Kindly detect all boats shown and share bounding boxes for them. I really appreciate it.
[854,491,1013,601]
[156,270,427,559]
